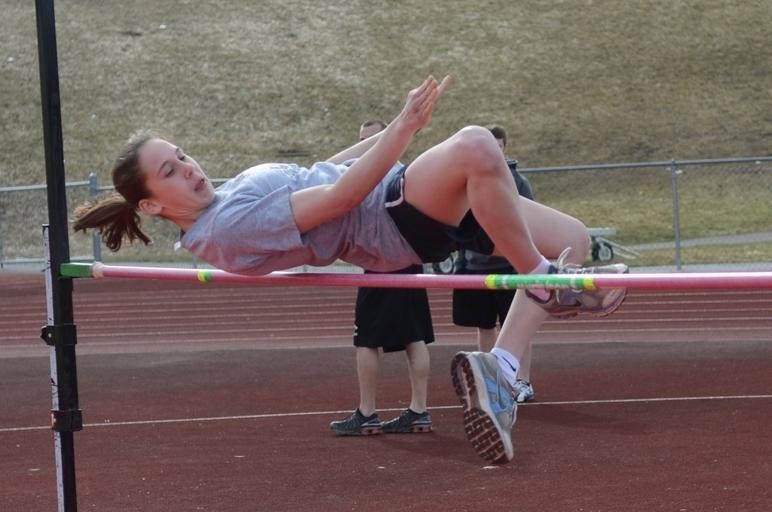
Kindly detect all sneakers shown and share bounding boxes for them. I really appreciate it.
[451,351,534,465]
[330,412,383,436]
[380,410,433,433]
[524,263,628,319]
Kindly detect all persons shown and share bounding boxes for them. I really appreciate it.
[452,125,535,402]
[71,74,630,466]
[329,118,434,436]
